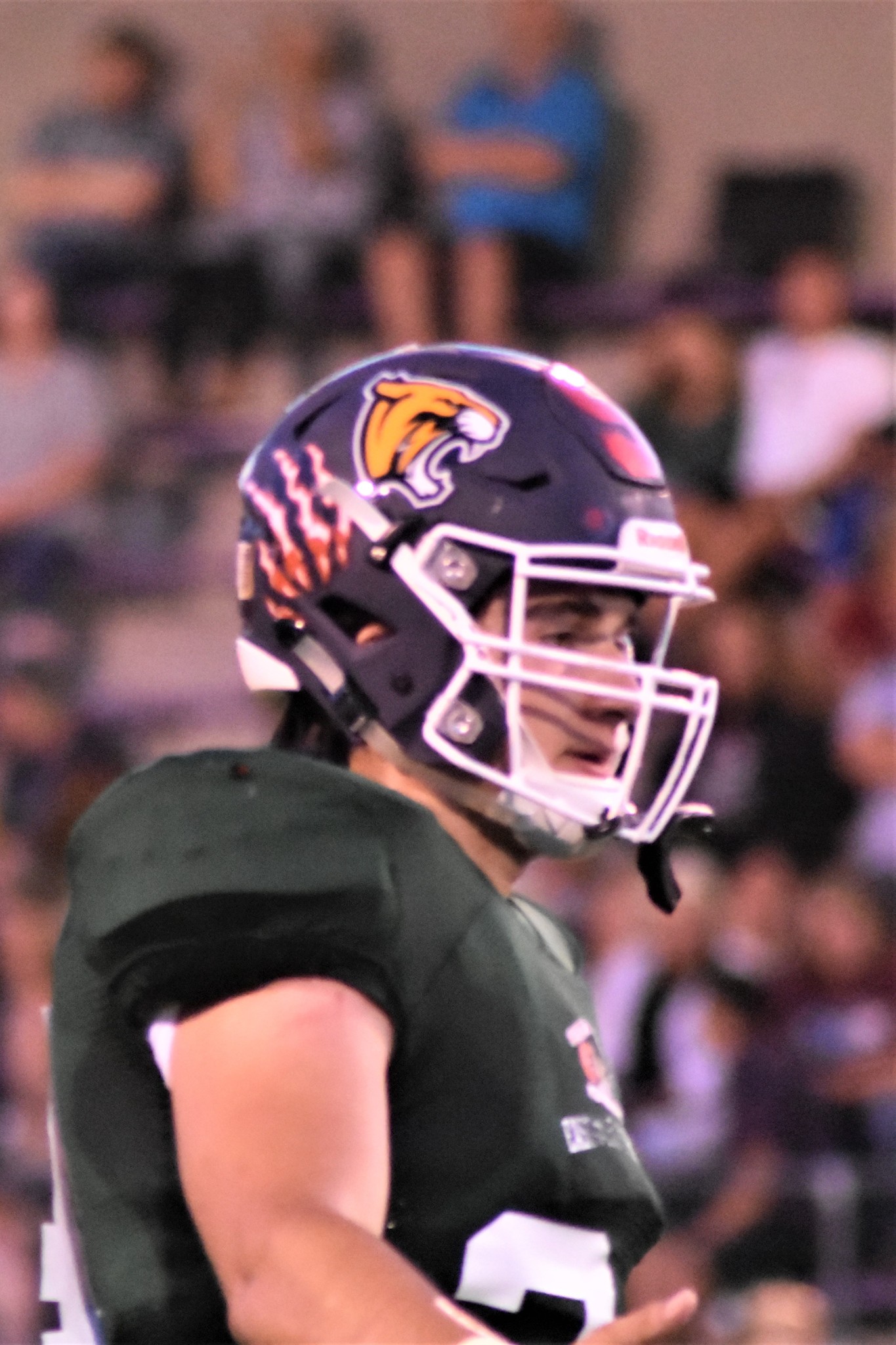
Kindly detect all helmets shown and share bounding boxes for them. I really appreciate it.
[231,339,724,859]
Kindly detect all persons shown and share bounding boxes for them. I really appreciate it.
[50,342,722,1345]
[0,0,896,1345]
